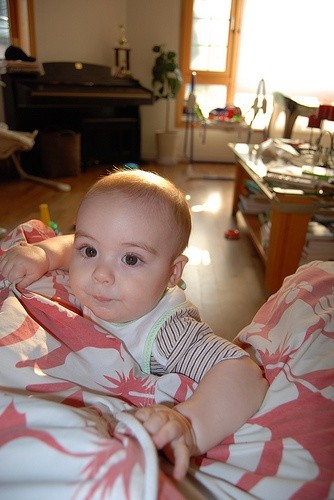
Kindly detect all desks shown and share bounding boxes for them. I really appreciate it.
[226,142,334,294]
[0,73,155,176]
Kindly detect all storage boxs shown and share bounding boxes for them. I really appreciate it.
[39,131,81,173]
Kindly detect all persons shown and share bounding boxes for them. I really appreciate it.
[0,169,269,482]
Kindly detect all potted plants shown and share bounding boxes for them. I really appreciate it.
[151,43,182,164]
